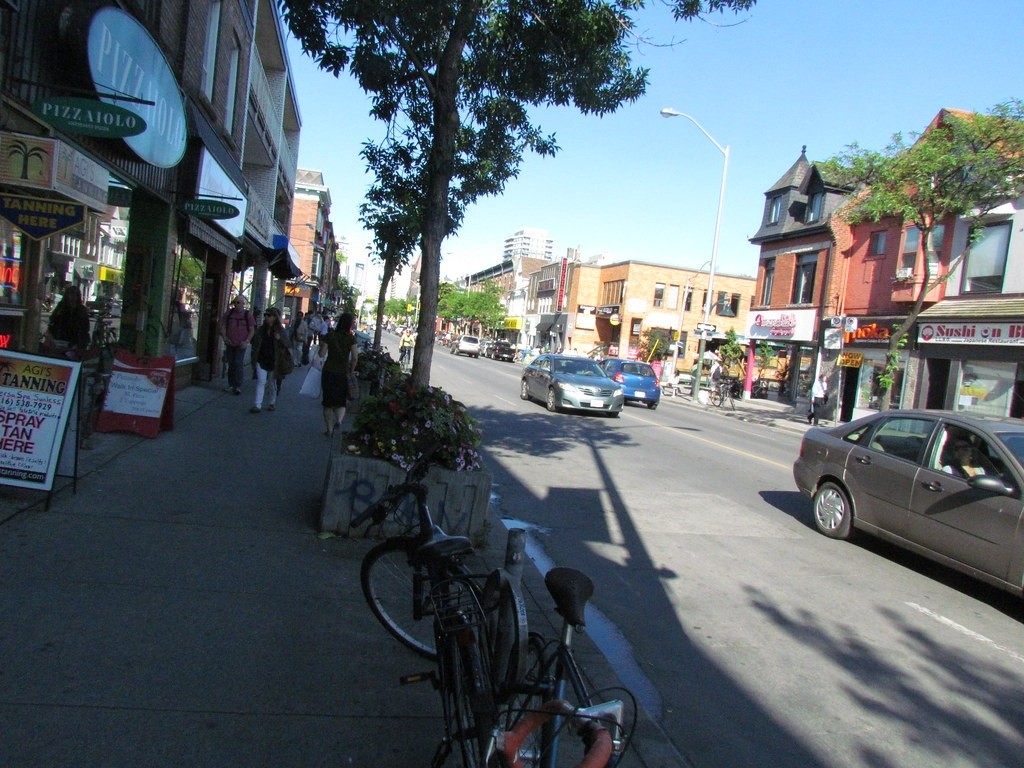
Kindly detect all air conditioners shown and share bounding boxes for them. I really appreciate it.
[896,267,913,279]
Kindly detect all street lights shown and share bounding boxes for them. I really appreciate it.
[448,252,472,298]
[659,105,730,404]
[671,261,712,375]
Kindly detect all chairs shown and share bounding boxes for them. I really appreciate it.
[940,434,999,477]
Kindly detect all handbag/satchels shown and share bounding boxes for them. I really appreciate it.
[279,348,294,375]
[347,372,359,401]
[295,333,306,342]
[299,352,322,399]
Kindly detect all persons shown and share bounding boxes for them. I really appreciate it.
[171,288,194,359]
[710,360,730,400]
[46,286,91,351]
[942,436,1005,480]
[690,360,698,397]
[808,375,827,427]
[778,365,790,392]
[318,313,357,436]
[289,311,332,367]
[220,295,288,413]
[399,330,415,369]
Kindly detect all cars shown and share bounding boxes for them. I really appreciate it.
[793,410,1024,602]
[478,340,493,356]
[394,325,419,339]
[520,354,625,418]
[598,357,661,410]
[450,335,480,358]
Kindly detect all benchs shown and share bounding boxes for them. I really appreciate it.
[877,434,926,462]
[661,384,681,398]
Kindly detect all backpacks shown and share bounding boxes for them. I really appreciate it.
[713,365,720,380]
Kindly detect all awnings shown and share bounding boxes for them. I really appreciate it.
[264,246,302,280]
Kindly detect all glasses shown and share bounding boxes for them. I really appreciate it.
[233,300,245,305]
[264,312,273,317]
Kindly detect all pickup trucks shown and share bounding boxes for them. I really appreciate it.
[485,342,516,362]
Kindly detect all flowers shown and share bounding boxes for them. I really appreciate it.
[347,380,486,474]
[344,345,402,379]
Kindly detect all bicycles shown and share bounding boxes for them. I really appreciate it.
[86,318,120,356]
[346,445,501,768]
[480,566,637,768]
[401,345,413,372]
[710,383,736,411]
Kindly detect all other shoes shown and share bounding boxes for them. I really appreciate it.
[251,407,259,413]
[333,423,341,431]
[269,404,276,410]
[324,430,332,438]
[224,386,234,392]
[234,388,241,394]
[807,416,812,424]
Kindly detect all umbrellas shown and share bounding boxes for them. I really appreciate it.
[695,350,722,361]
